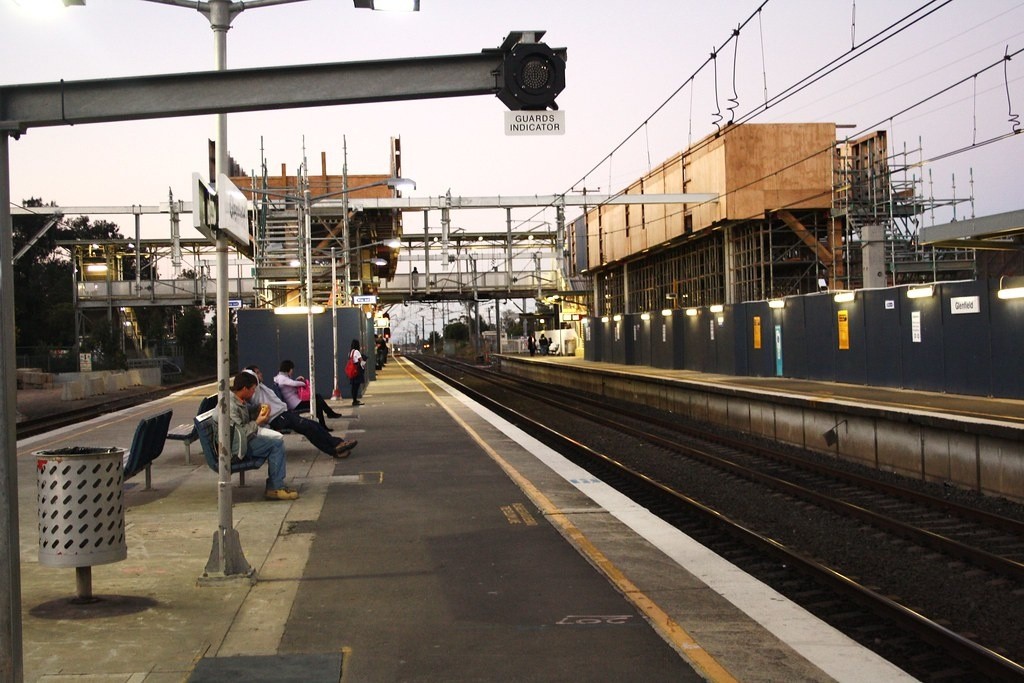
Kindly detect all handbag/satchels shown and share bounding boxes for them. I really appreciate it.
[298,378,310,401]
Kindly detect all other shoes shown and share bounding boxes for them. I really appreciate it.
[334,439,358,458]
[352,401,365,405]
[320,424,334,432]
[263,488,298,500]
[327,410,342,419]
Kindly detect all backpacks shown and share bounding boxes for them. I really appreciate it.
[345,349,358,378]
[211,417,247,465]
[267,382,283,402]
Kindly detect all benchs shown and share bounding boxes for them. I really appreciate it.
[122,407,173,492]
[193,406,268,488]
[167,392,218,466]
[273,383,311,415]
[537,343,559,355]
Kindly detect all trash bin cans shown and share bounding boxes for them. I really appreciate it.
[33,447,128,567]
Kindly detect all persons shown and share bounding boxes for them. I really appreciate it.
[212,372,298,500]
[528,333,537,356]
[273,360,342,432]
[548,338,552,346]
[374,334,389,375]
[243,365,358,458]
[539,334,549,356]
[344,340,367,405]
